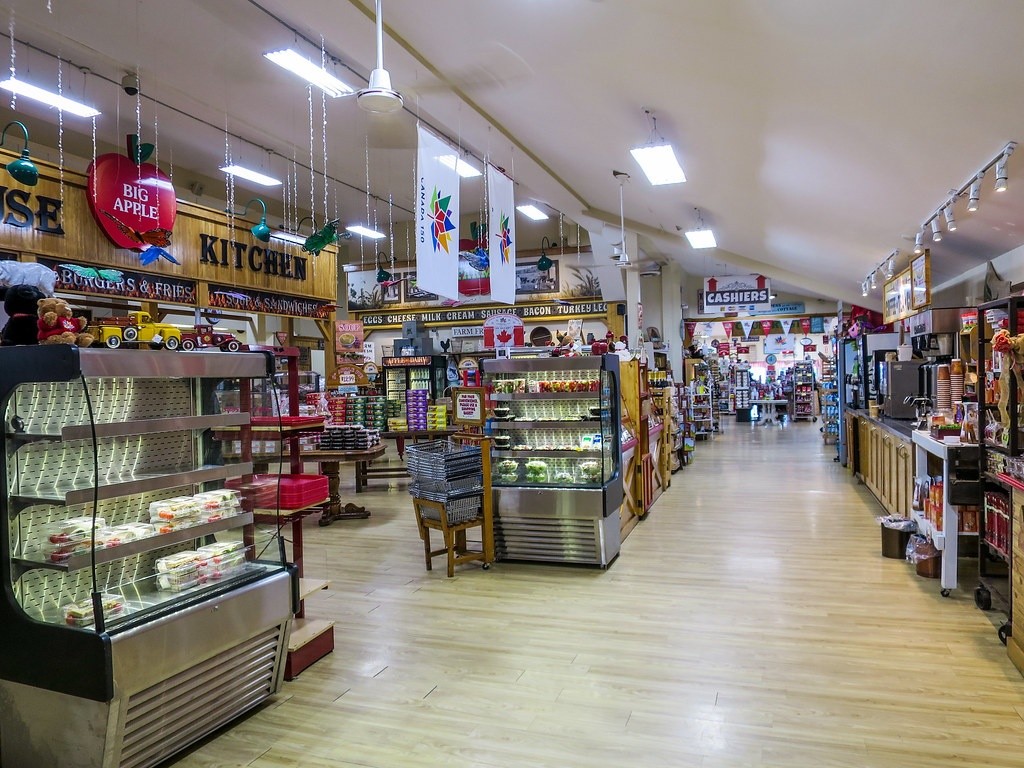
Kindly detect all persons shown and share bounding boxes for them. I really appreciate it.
[0,286,44,346]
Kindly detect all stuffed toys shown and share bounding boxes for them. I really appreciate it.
[615,341,630,360]
[560,337,583,356]
[37,297,94,348]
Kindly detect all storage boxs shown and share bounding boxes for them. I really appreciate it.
[394,321,433,357]
[306,389,448,432]
[985,308,1024,334]
[924,482,980,532]
[931,425,961,440]
[233,437,317,454]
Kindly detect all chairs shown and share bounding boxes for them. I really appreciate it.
[413,497,485,578]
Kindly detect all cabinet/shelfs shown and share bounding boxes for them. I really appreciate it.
[348,300,627,387]
[620,345,839,544]
[215,344,336,682]
[843,410,973,520]
[973,295,1024,645]
[912,428,991,597]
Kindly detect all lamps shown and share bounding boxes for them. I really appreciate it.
[861,147,1013,298]
[1,0,717,250]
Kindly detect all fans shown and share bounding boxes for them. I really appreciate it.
[332,0,510,150]
[565,174,665,269]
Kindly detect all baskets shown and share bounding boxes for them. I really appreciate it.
[404,438,482,526]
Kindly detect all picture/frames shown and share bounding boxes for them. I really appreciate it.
[403,260,559,302]
[381,273,401,303]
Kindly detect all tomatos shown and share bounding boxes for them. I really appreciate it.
[196,558,222,582]
[66,615,74,624]
[204,501,222,523]
[158,511,174,534]
[114,603,122,612]
[49,533,72,562]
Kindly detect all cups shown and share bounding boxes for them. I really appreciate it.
[871,406,879,418]
[936,359,964,409]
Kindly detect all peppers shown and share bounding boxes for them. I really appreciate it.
[539,380,600,393]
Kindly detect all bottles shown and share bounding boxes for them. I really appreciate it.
[410,369,430,390]
[647,370,673,420]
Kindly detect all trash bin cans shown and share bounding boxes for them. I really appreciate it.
[913,534,942,578]
[880,517,916,560]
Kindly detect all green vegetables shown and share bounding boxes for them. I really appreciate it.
[498,460,601,484]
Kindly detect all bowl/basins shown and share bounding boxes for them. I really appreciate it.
[588,407,607,422]
[498,458,602,485]
[493,409,515,450]
[37,489,249,628]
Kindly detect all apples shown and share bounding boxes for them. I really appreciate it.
[592,342,607,355]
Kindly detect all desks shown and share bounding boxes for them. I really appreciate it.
[748,400,789,426]
[356,426,463,493]
[222,444,389,520]
